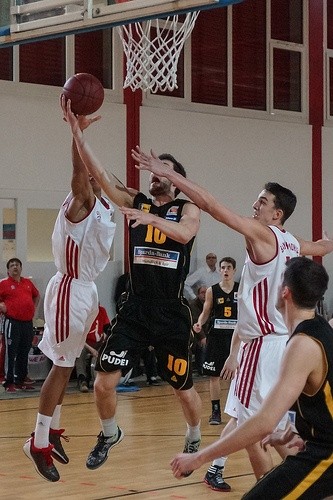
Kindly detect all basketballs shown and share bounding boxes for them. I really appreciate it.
[62,73,105,117]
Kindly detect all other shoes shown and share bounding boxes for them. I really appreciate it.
[129,382,135,388]
[146,380,162,386]
[15,384,35,391]
[24,377,36,384]
[79,381,88,392]
[5,383,16,393]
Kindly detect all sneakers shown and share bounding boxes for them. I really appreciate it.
[180,436,202,477]
[30,427,70,465]
[203,465,232,492]
[23,437,61,482]
[86,425,125,470]
[209,409,221,425]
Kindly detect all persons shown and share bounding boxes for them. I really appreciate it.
[182,252,223,301]
[193,257,239,424]
[167,257,333,500]
[189,287,207,344]
[21,115,116,483]
[0,258,40,394]
[59,91,200,478]
[130,145,332,484]
[75,302,110,392]
